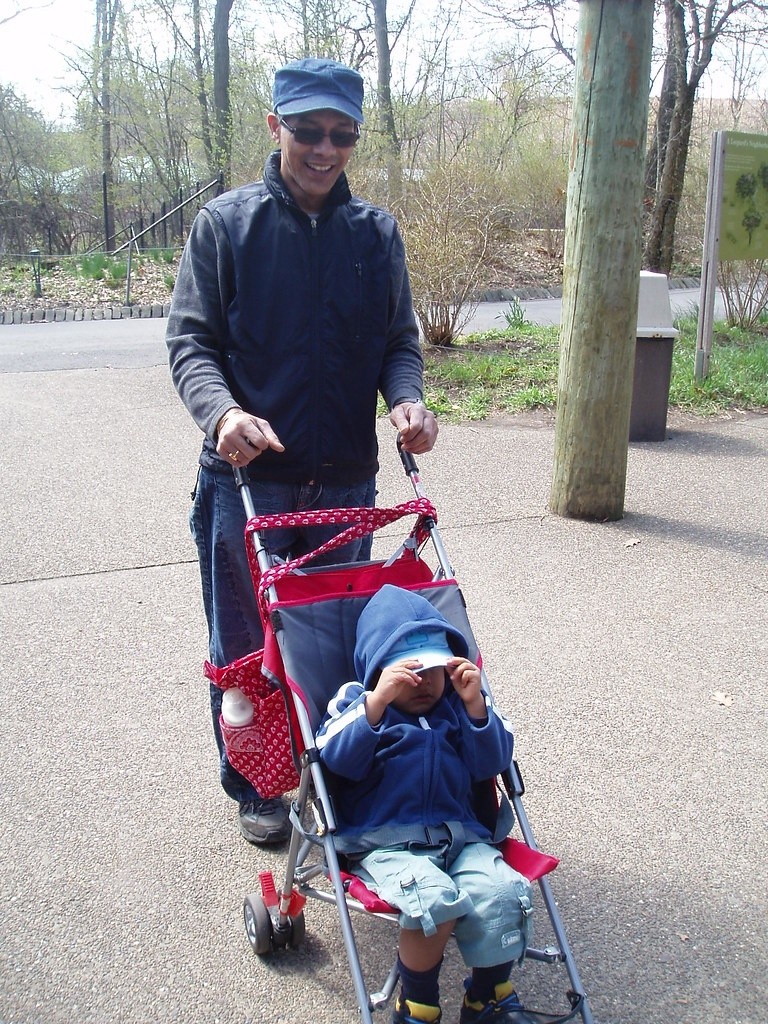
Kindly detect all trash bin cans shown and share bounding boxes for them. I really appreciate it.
[630,270,679,442]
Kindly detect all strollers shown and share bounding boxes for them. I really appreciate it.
[201,432,596,1024]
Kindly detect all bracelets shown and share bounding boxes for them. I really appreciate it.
[216,409,244,435]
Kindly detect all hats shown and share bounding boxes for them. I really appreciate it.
[274,59,364,124]
[379,628,456,674]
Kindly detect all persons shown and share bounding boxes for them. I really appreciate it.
[165,63,442,847]
[315,584,542,1024]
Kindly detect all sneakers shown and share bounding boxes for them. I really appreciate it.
[239,794,290,844]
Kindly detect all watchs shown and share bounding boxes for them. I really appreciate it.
[394,398,428,410]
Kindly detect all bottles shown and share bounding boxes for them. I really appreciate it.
[220,687,253,727]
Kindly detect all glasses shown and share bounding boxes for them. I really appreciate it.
[280,120,360,147]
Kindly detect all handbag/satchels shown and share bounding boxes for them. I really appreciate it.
[203,645,299,798]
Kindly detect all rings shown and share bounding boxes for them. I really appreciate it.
[228,451,240,462]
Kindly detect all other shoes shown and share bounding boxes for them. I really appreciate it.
[393,1000,443,1024]
[460,977,542,1024]
